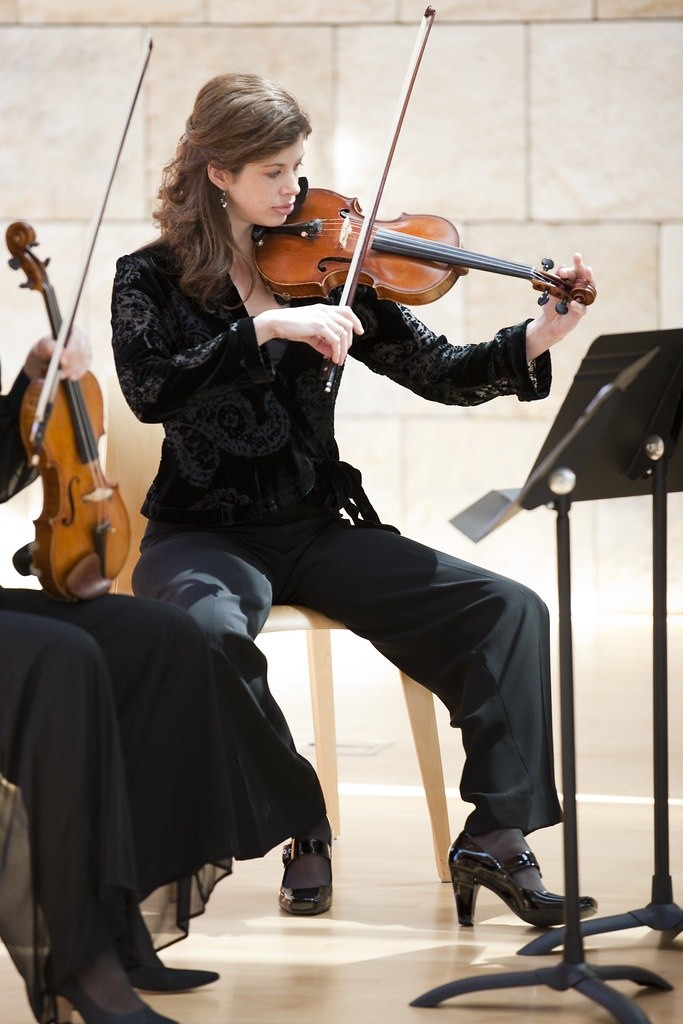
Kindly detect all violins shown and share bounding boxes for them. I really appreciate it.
[253,188,598,316]
[3,221,133,603]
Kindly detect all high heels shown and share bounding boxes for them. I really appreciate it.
[43,950,181,1024]
[279,815,332,915]
[105,922,220,993]
[448,830,598,926]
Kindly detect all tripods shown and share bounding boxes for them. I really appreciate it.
[408,333,683,1024]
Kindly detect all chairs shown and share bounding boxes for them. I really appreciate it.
[105,377,451,883]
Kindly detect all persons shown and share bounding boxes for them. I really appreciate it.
[0,321,237,1023]
[109,69,601,928]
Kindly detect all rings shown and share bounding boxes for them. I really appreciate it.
[335,326,345,337]
